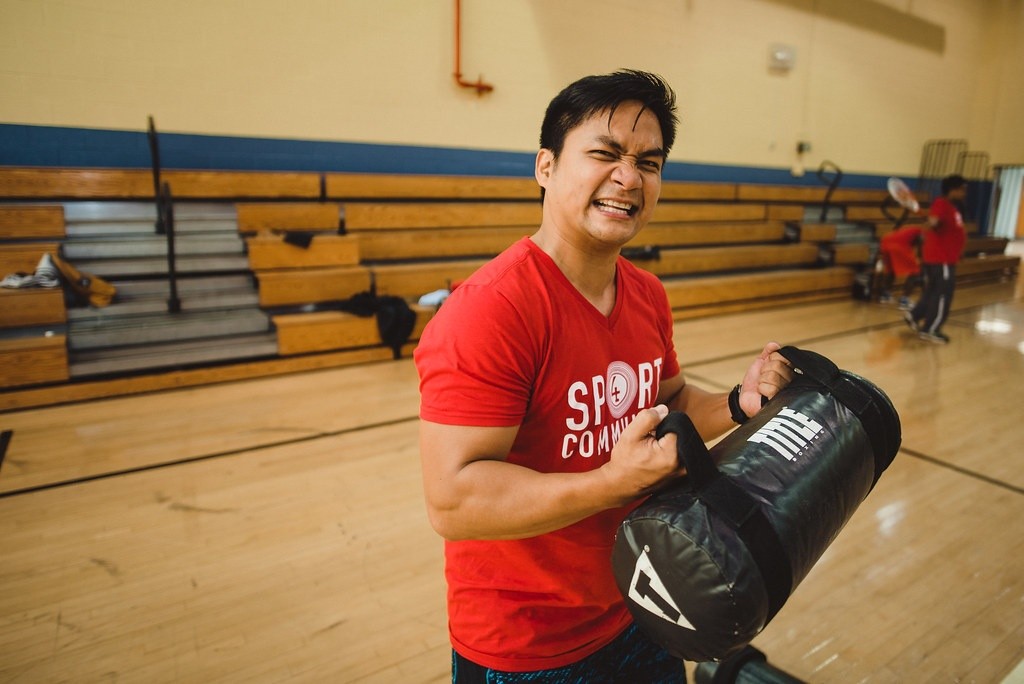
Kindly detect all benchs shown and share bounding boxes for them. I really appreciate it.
[0,167,1022,413]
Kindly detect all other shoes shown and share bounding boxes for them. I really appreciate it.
[906,309,920,334]
[897,297,915,310]
[919,326,950,345]
[877,289,893,306]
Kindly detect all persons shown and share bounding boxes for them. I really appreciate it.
[905,174,968,346]
[413,68,797,684]
[879,224,927,312]
